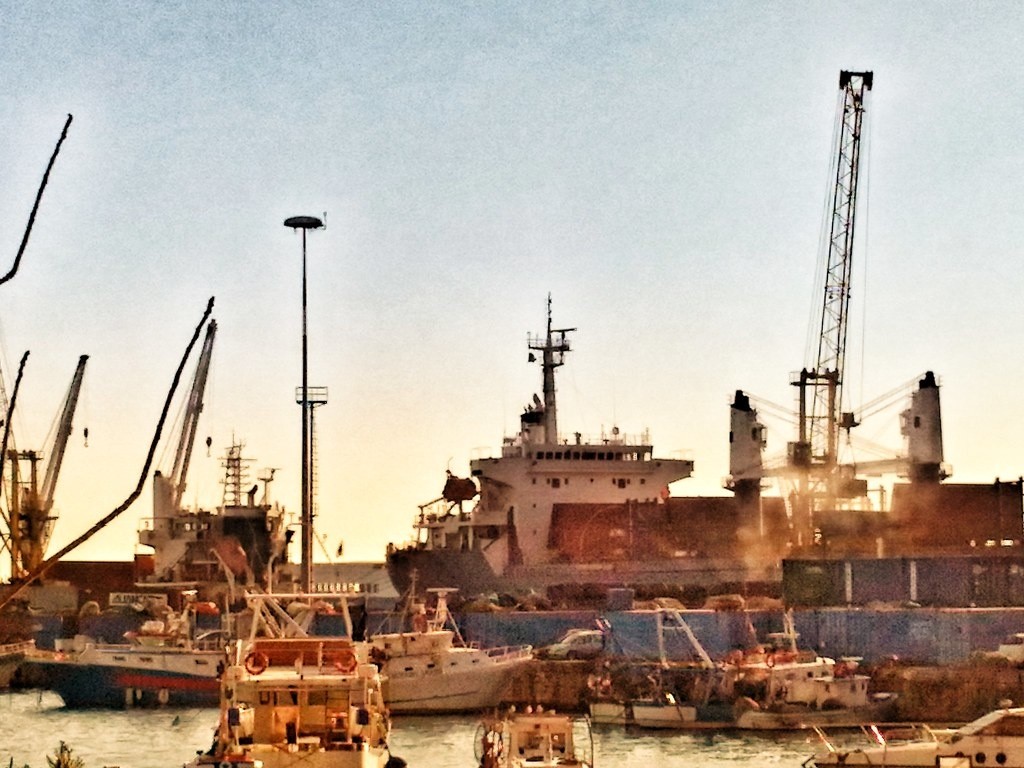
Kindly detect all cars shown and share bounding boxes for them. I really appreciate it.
[549,630,602,659]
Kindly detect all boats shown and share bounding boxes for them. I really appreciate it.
[355,584,532,711]
[584,602,891,729]
[481,709,592,768]
[384,290,1023,656]
[185,590,406,768]
[24,594,227,706]
[814,705,1024,768]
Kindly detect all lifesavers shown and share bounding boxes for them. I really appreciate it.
[766,654,776,668]
[332,651,358,672]
[245,652,270,676]
